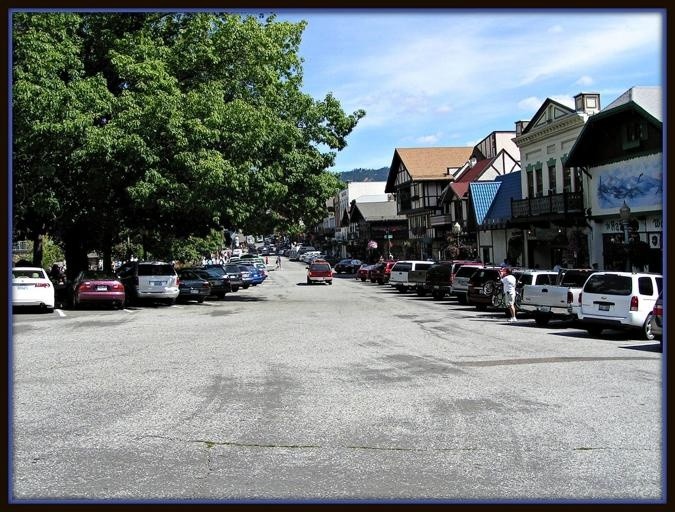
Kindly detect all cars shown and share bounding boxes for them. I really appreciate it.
[177,234,365,307]
[71,270,125,307]
[11,266,56,314]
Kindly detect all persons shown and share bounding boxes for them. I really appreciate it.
[499,269,518,322]
[224,252,227,260]
[277,254,281,269]
[227,251,230,257]
[554,262,562,272]
[500,258,511,267]
[265,256,268,264]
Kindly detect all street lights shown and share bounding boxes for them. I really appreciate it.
[454,221,463,253]
[619,200,633,273]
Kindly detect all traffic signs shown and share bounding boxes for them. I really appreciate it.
[384,235,393,239]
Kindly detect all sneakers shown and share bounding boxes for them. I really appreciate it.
[507,316,519,322]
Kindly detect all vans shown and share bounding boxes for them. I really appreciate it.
[116,261,180,304]
